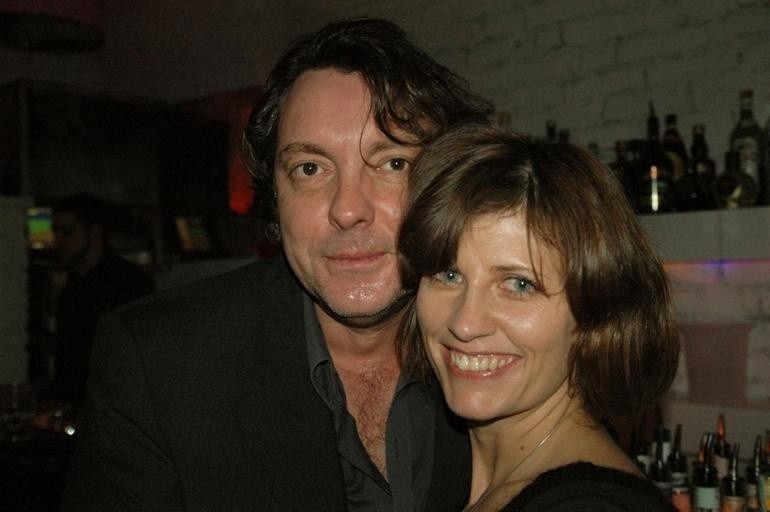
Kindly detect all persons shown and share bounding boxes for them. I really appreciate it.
[398,123,680,512]
[88,19,495,512]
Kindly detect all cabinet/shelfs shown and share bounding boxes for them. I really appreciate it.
[6,81,258,214]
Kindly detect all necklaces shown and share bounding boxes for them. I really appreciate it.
[470,404,582,512]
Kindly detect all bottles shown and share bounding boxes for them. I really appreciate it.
[646,414,769,512]
[640,90,763,210]
